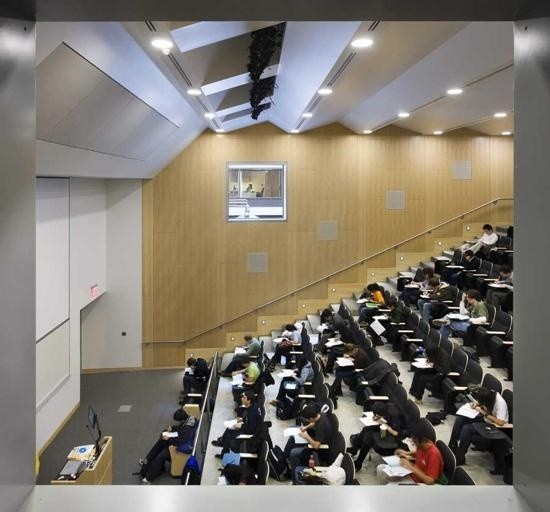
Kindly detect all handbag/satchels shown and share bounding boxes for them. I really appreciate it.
[268,445,290,482]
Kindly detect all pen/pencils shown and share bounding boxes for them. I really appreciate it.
[301,422,305,426]
[399,484,419,486]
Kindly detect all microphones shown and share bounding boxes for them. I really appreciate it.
[85,424,98,443]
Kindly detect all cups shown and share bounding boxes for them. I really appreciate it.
[380,425,387,438]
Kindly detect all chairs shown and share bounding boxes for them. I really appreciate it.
[169,226,513,485]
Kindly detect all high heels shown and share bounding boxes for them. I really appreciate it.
[347,447,362,473]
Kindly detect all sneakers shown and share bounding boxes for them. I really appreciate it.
[331,387,343,396]
[212,440,222,446]
[410,388,422,400]
[218,370,232,377]
[433,319,449,327]
[373,340,384,346]
[216,454,221,459]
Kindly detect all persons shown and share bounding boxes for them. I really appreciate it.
[181,357,209,394]
[441,249,462,283]
[399,265,513,338]
[316,283,513,485]
[449,250,479,287]
[460,223,499,255]
[138,408,198,483]
[212,324,346,484]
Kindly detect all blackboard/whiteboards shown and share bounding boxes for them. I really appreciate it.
[36,321,70,412]
